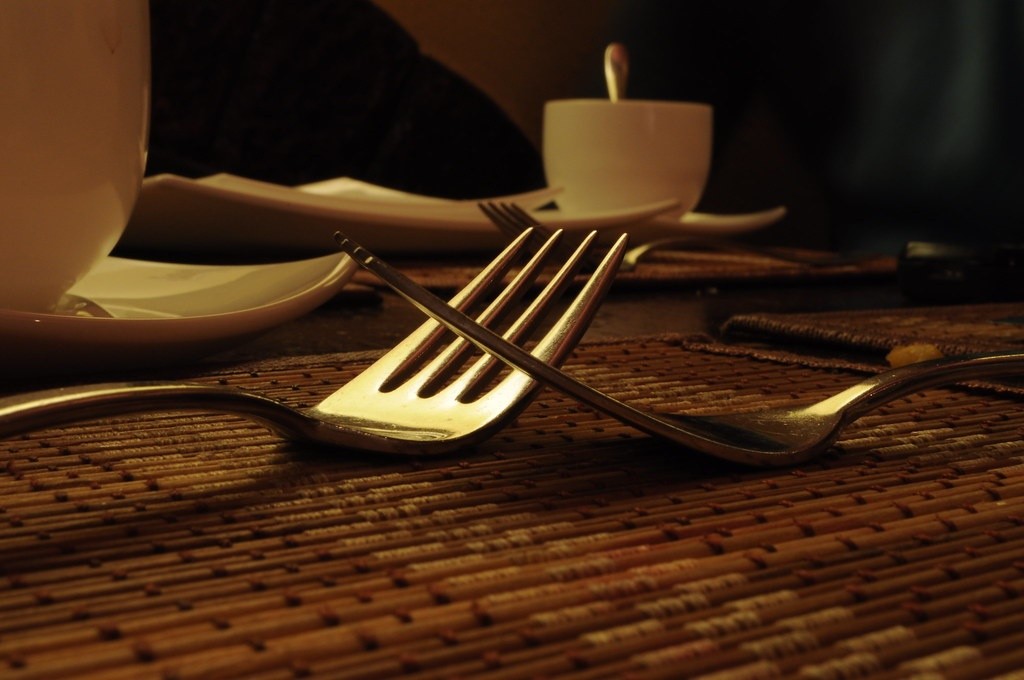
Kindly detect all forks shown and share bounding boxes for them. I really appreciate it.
[0,225,632,456]
[477,199,690,271]
[333,228,1024,469]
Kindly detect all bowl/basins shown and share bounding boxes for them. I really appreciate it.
[544,99,715,216]
[0,1,149,314]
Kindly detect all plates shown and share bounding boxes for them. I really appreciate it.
[0,249,356,342]
[126,174,789,253]
